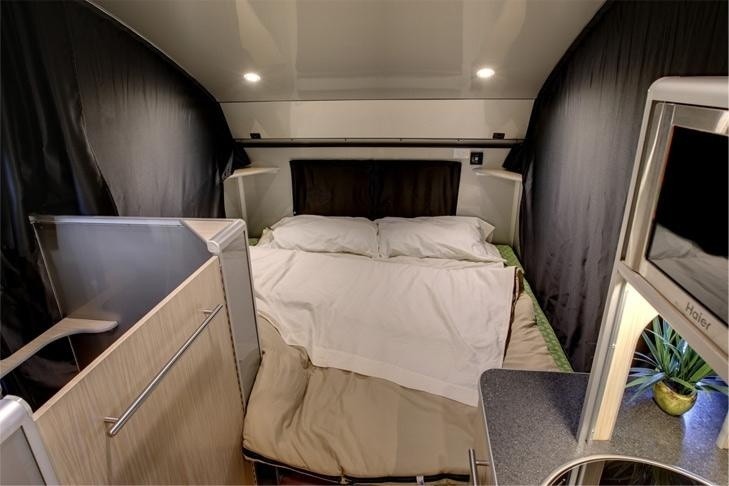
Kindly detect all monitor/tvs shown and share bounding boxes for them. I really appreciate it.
[624,102,729,359]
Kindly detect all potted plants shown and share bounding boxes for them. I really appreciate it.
[624,314,729,414]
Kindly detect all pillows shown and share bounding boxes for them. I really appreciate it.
[257,215,509,265]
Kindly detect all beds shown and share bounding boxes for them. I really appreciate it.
[241,235,577,486]
